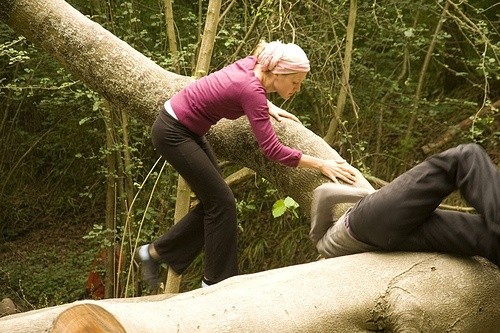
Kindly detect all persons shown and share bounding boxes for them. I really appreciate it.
[309,142,500,272]
[132,41,357,292]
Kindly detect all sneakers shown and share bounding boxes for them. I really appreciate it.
[135,244,160,290]
[201,275,217,288]
